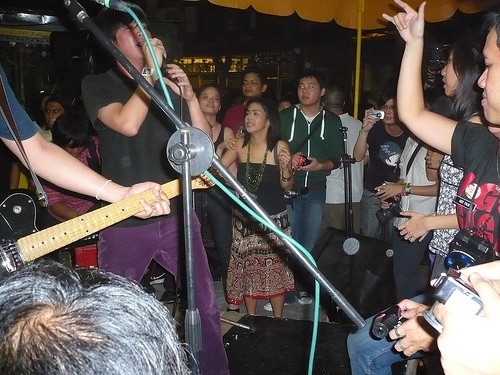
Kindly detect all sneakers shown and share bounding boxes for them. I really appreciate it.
[296,290,312,304]
[264,302,273,311]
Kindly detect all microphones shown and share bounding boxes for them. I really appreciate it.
[161,56,181,87]
[94,0,130,13]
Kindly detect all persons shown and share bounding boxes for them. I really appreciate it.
[382,0,500,260]
[0,0,500,375]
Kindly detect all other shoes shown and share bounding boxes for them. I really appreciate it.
[158,293,182,304]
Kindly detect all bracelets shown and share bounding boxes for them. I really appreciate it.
[282,170,292,182]
[402,182,411,196]
[96,179,112,200]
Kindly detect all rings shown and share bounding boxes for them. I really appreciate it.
[394,327,403,338]
[229,139,231,142]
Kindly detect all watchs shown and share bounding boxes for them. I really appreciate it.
[141,67,159,80]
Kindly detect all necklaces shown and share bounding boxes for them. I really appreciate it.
[245,139,269,193]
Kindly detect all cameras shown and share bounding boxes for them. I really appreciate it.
[423,272,485,334]
[375,196,405,225]
[369,111,385,119]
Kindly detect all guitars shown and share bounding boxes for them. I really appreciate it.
[0,172,217,287]
